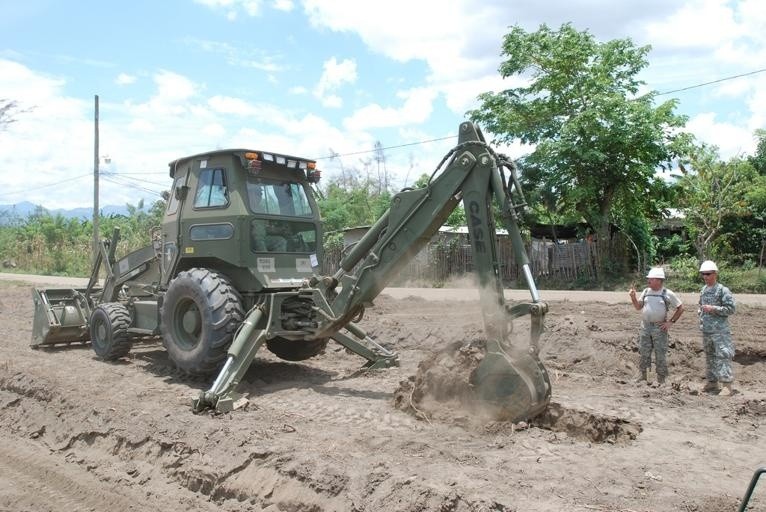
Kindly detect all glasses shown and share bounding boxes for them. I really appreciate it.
[703,273,713,275]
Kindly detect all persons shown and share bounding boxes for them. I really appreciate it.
[629,267,685,384]
[247,182,292,253]
[696,259,737,396]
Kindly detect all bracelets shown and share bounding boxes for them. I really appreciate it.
[671,319,676,323]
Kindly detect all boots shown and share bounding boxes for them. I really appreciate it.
[638,373,647,381]
[702,379,718,392]
[658,377,665,384]
[719,382,733,396]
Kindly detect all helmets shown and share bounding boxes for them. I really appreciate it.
[699,261,718,272]
[646,267,665,279]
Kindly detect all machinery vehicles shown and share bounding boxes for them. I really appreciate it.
[32,121,553,429]
